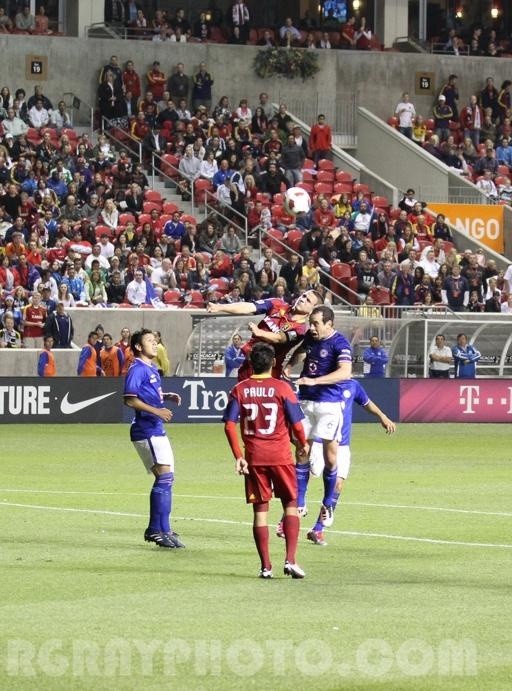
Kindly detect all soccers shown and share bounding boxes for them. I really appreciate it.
[284,186,311,215]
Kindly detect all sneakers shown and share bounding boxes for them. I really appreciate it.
[144,528,185,548]
[284,560,306,578]
[258,565,273,578]
[276,502,333,545]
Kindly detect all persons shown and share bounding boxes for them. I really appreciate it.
[1,0,512,380]
[123,328,186,548]
[280,306,353,528]
[277,379,396,546]
[221,343,311,579]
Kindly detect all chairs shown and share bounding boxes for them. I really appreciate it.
[0,23,70,38]
[186,22,399,51]
